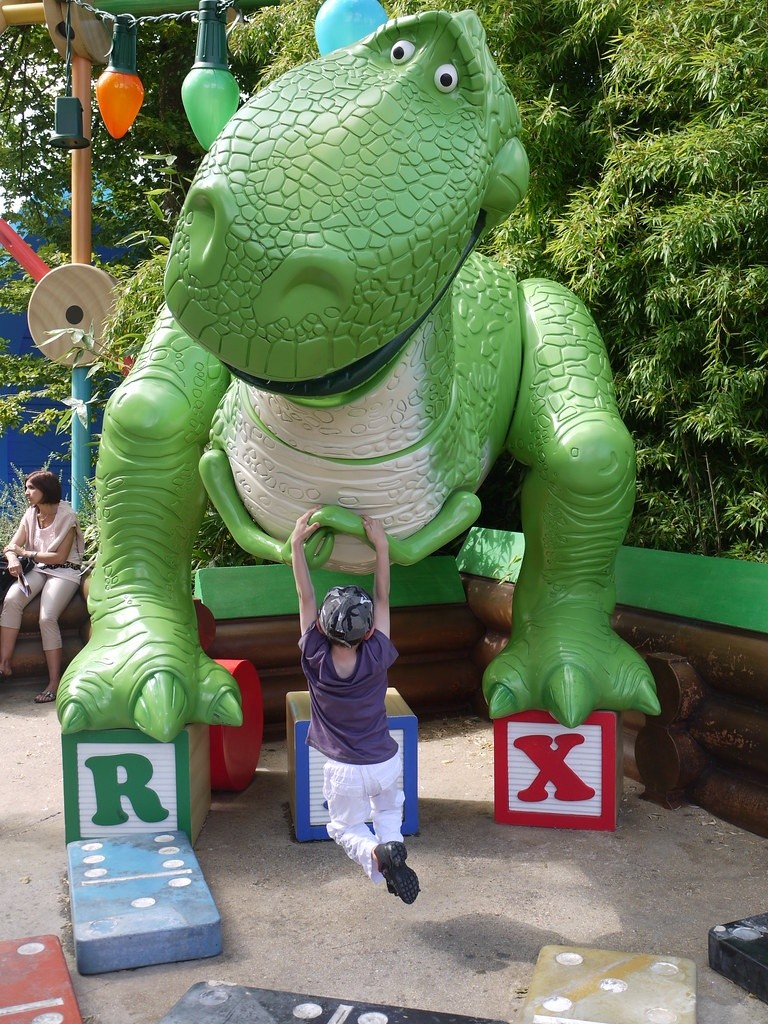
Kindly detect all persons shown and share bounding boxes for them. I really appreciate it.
[0,472,84,703]
[291,504,419,904]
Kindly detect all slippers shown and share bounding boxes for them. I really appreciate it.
[0,671,6,682]
[35,691,56,703]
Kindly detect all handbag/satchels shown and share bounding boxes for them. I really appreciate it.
[0,555,35,603]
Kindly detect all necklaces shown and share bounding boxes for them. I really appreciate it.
[38,513,50,522]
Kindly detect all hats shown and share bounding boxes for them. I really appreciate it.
[320,585,373,648]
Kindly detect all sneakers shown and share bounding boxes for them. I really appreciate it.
[375,841,420,904]
